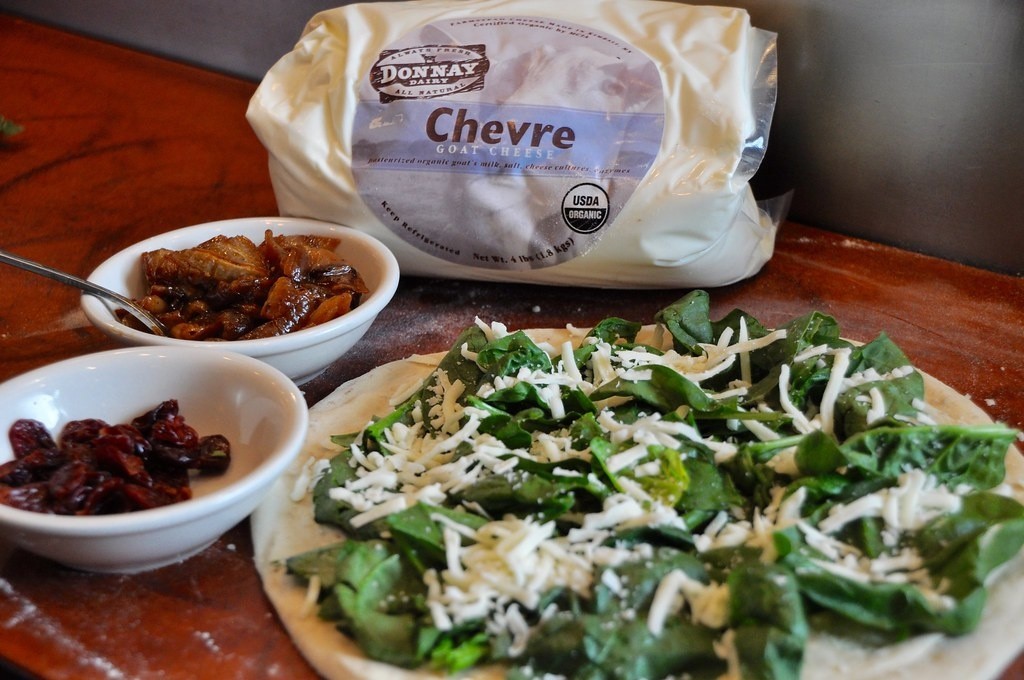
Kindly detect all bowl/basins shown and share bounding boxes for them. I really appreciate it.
[0,345,309,572]
[82,217,400,387]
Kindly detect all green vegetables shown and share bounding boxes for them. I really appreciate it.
[264,290,1024,680]
